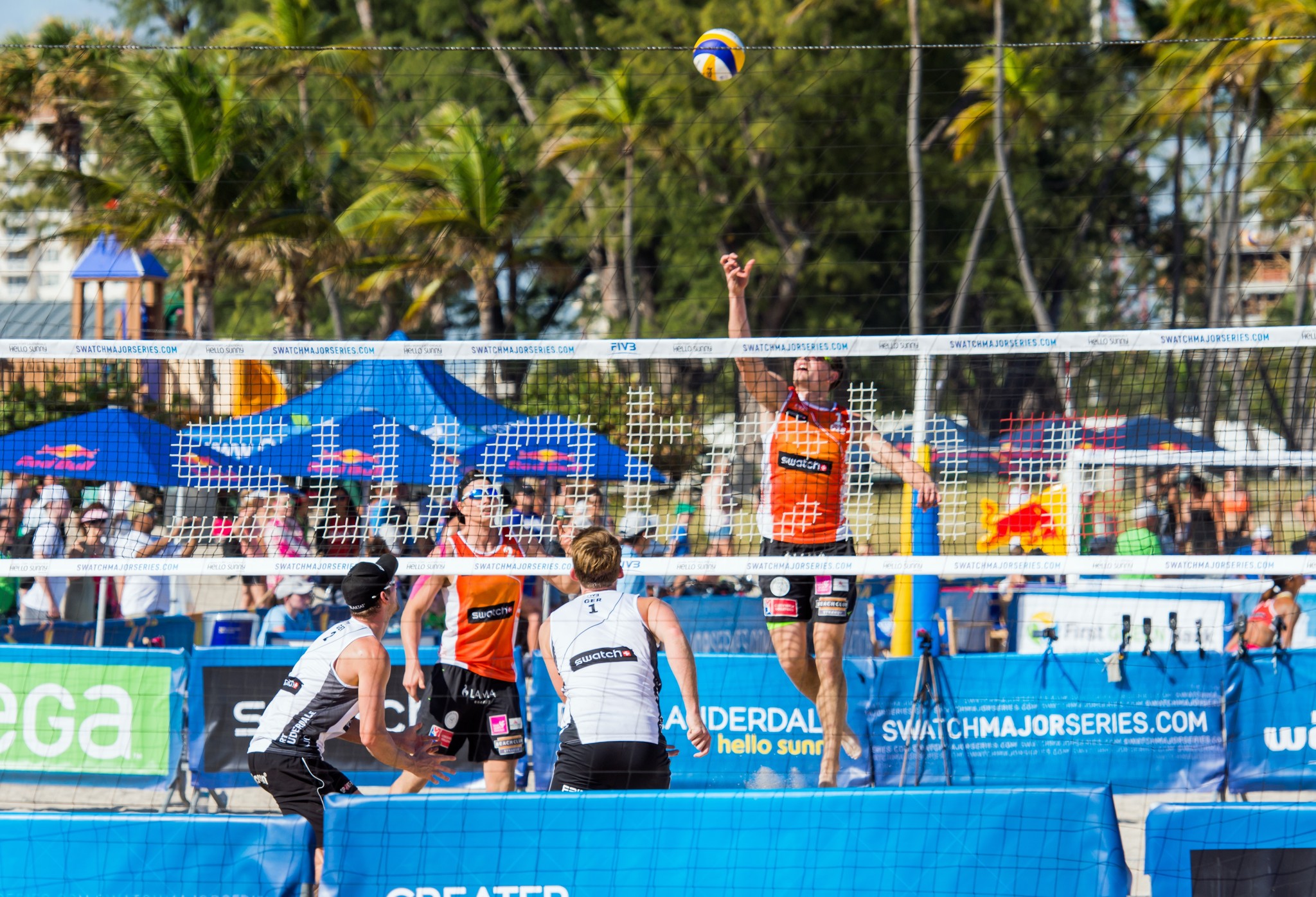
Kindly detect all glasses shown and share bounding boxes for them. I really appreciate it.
[377,574,396,598]
[90,519,106,523]
[796,356,836,365]
[461,488,498,501]
[334,496,346,501]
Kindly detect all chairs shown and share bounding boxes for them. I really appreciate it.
[867,602,1009,656]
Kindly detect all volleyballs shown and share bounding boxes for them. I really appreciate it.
[692,27,746,82]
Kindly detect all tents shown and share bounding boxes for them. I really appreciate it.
[0,327,1230,489]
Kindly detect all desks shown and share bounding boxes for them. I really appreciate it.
[265,631,442,647]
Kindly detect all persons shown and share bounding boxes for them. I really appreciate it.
[719,251,942,787]
[0,455,1316,897]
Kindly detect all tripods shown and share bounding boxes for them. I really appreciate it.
[898,629,953,786]
[156,646,227,816]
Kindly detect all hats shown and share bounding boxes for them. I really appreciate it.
[341,554,399,613]
[520,482,535,494]
[274,576,317,599]
[81,508,108,524]
[617,511,661,539]
[33,484,69,507]
[127,500,155,521]
[1253,525,1272,540]
[675,503,696,515]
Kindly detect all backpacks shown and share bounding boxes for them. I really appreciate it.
[10,522,62,587]
